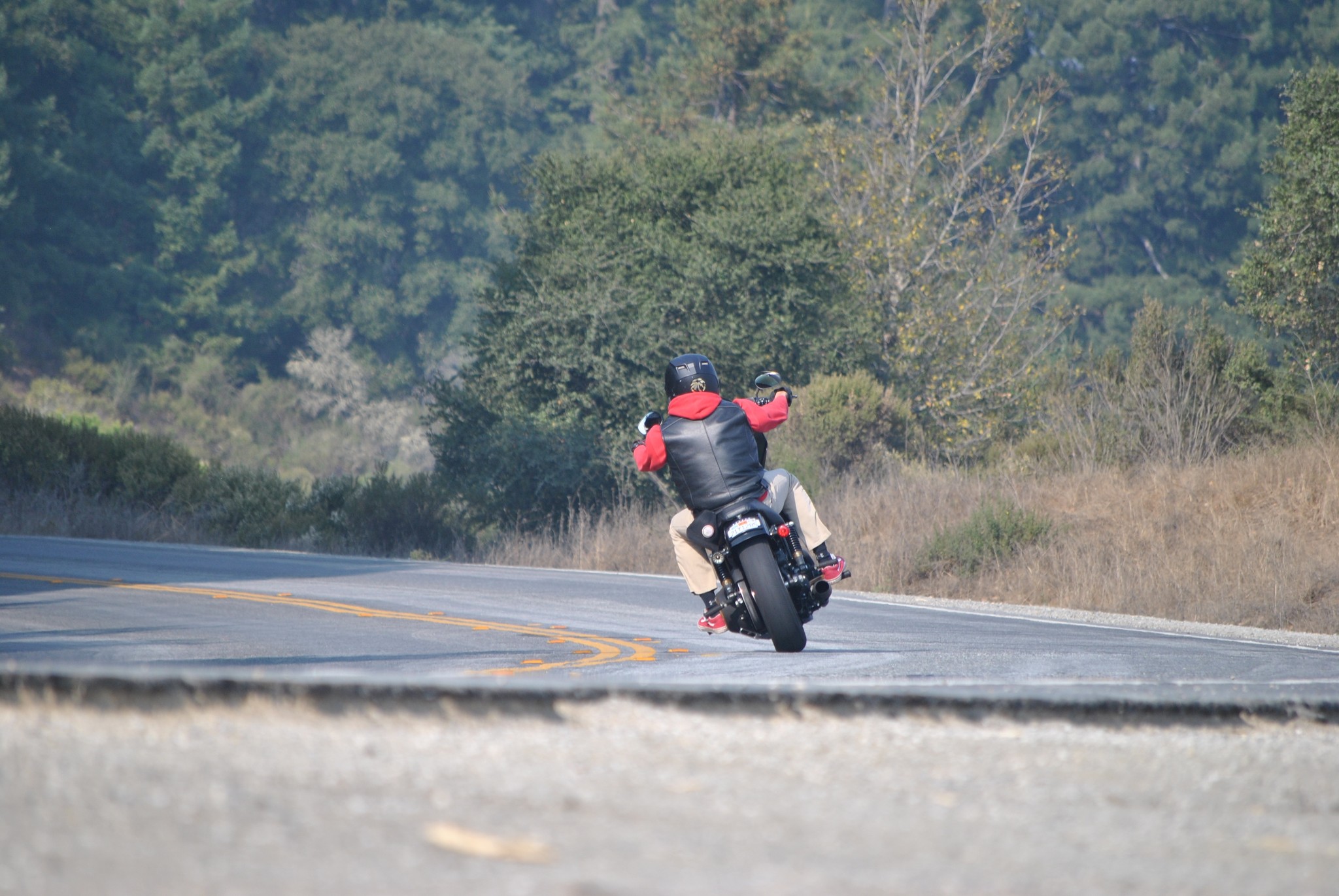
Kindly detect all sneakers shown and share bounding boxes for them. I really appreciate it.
[697,608,727,634]
[821,556,846,585]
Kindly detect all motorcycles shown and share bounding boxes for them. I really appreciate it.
[636,372,852,652]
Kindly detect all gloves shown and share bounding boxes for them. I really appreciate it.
[770,387,792,406]
[631,439,646,452]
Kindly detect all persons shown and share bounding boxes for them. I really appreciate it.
[629,353,847,634]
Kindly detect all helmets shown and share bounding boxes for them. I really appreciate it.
[665,353,719,404]
[644,411,661,431]
